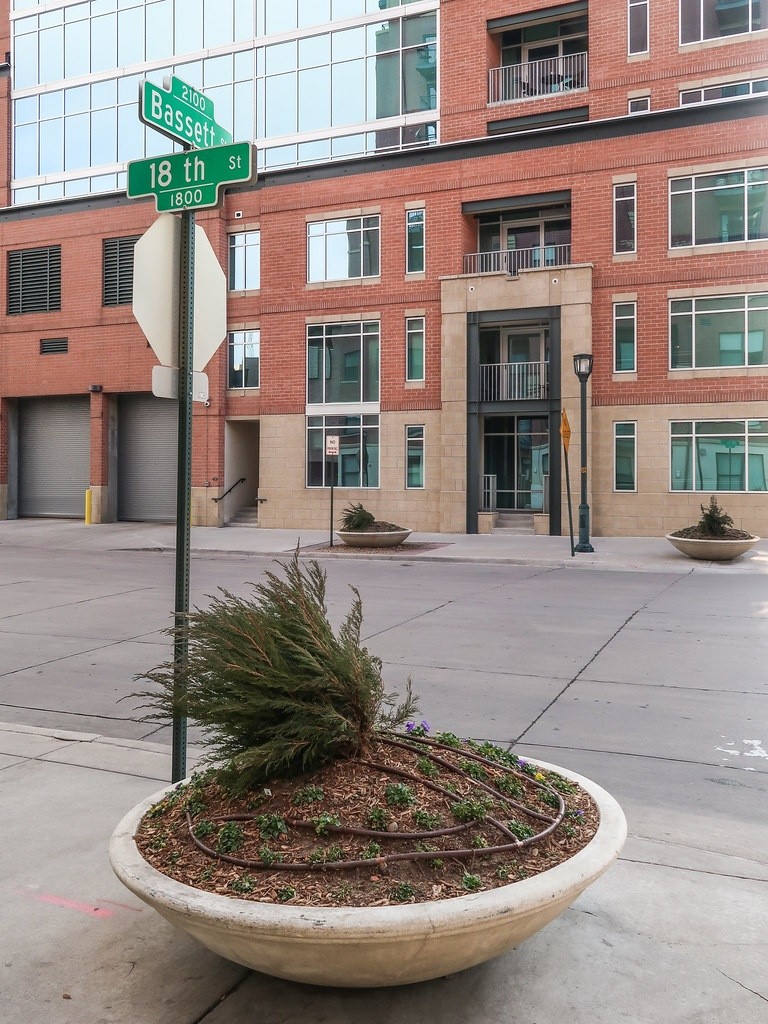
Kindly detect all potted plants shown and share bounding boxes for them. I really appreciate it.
[664,492,760,561]
[336,499,413,547]
[95,536,628,990]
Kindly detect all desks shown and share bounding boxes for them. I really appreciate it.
[541,74,564,95]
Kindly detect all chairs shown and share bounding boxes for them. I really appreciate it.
[565,71,585,90]
[516,78,541,96]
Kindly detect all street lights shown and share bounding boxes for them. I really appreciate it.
[572,352,595,552]
